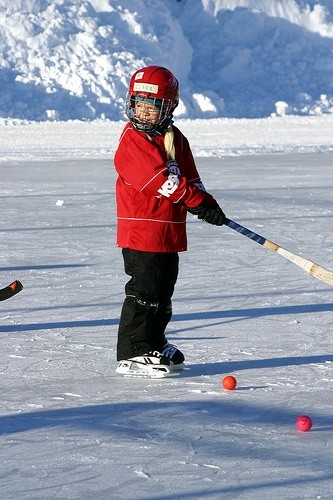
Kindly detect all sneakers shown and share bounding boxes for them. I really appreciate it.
[116,350,174,379]
[159,341,186,370]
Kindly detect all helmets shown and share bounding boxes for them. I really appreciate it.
[129,65,179,113]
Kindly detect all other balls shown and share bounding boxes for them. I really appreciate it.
[223,376,236,390]
[296,414,312,430]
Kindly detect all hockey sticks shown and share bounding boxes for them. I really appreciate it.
[221,217,332,287]
[0,279,24,301]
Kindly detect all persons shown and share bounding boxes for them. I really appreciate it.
[113,66,226,375]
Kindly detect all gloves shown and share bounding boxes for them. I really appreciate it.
[187,192,225,226]
